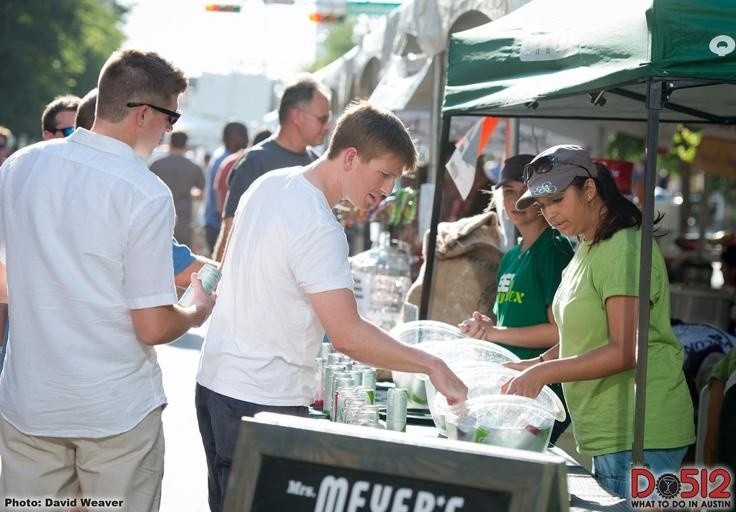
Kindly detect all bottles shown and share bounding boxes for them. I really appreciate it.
[345,226,412,329]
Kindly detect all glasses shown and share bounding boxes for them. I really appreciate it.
[520,154,593,184]
[45,126,75,137]
[289,104,328,127]
[123,101,184,127]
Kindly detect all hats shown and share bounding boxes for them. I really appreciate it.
[511,140,601,209]
[496,152,538,190]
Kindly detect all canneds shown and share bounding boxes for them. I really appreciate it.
[309,342,408,433]
[178,263,223,309]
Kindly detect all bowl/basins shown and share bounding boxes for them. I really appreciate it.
[387,320,556,452]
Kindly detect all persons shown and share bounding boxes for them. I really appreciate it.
[2,48,735,511]
